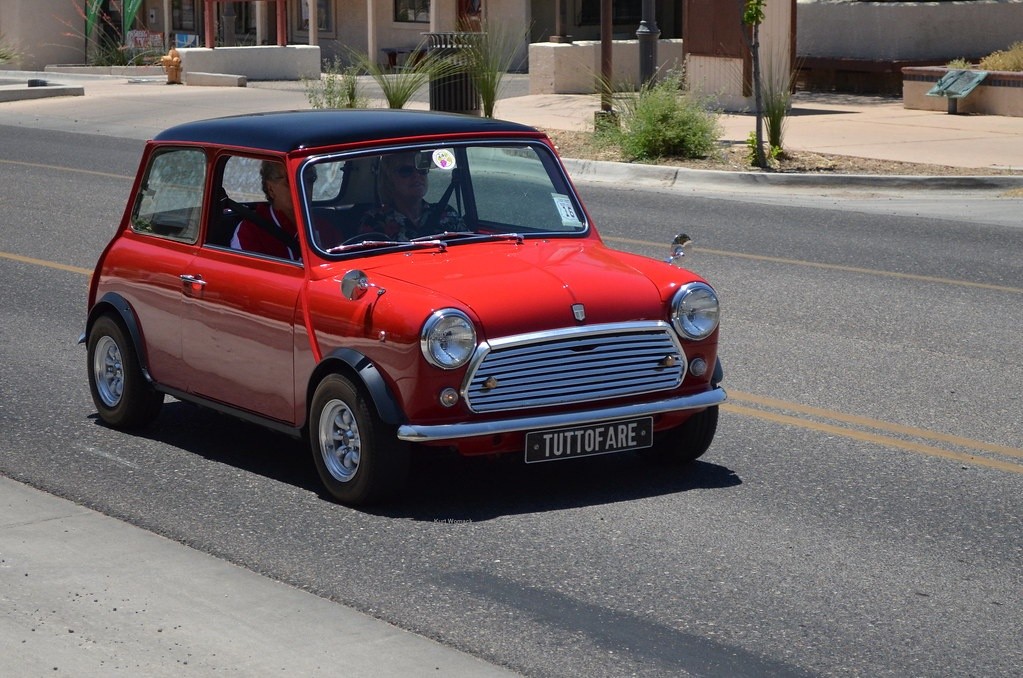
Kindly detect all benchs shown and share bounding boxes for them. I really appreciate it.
[213,203,386,249]
[381,47,428,72]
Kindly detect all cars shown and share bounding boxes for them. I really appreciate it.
[77,106,728,507]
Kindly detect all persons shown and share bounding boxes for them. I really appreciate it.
[229,159,346,262]
[359,150,470,245]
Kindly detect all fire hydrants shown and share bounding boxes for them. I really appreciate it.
[160,45,184,85]
